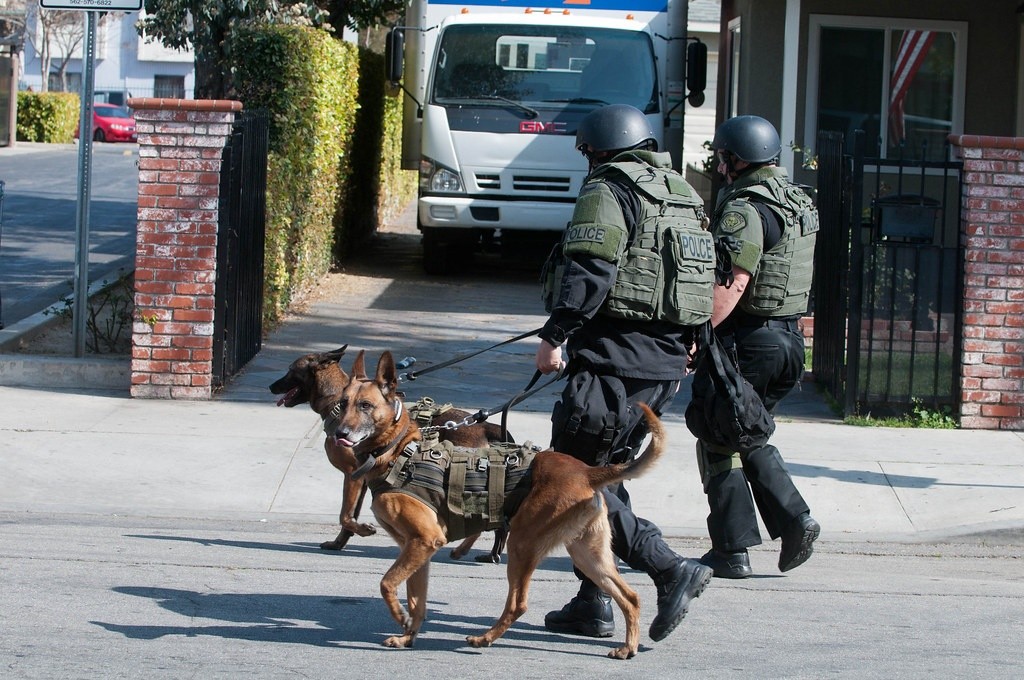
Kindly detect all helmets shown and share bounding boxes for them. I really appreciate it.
[574,102,659,152]
[712,114,781,163]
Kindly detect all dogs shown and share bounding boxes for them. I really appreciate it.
[269,344,516,566]
[335,349,666,660]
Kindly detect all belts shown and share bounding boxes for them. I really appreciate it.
[762,319,797,329]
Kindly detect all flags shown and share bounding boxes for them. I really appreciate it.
[890,30,937,145]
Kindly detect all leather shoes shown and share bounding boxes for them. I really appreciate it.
[544,579,614,636]
[650,556,714,642]
[691,545,752,580]
[778,512,820,572]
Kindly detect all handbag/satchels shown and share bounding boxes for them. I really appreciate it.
[686,326,775,453]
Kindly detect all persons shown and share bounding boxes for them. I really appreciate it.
[684,115,820,577]
[536,104,713,642]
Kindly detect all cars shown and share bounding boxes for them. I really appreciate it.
[74,104,137,144]
[93,90,130,113]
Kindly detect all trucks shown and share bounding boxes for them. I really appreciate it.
[387,1,708,276]
